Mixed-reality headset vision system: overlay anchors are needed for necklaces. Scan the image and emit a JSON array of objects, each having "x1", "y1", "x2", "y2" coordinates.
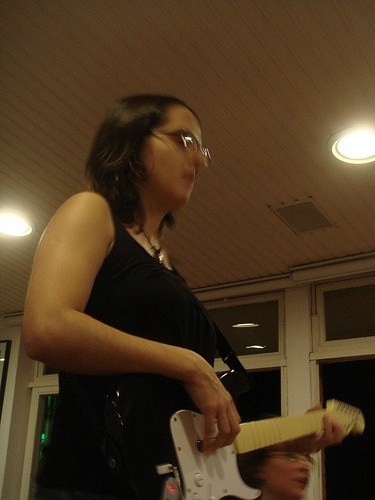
[{"x1": 143, "y1": 232, "x2": 164, "y2": 265}]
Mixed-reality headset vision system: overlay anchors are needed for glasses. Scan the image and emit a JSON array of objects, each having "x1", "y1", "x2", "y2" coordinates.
[
  {"x1": 153, "y1": 127, "x2": 211, "y2": 177},
  {"x1": 271, "y1": 451, "x2": 315, "y2": 466}
]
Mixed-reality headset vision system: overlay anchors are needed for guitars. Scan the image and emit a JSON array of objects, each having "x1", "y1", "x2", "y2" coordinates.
[{"x1": 157, "y1": 399, "x2": 366, "y2": 500}]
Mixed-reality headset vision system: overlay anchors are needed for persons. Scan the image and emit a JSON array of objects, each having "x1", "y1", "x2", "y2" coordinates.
[
  {"x1": 219, "y1": 431, "x2": 315, "y2": 500},
  {"x1": 21, "y1": 91, "x2": 343, "y2": 500}
]
